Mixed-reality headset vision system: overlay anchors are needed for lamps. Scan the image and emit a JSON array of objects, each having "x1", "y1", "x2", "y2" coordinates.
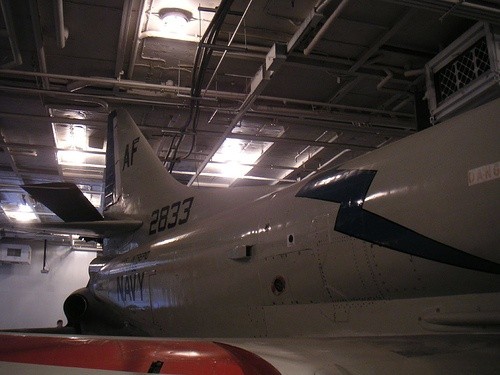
[{"x1": 157, "y1": 7, "x2": 192, "y2": 34}]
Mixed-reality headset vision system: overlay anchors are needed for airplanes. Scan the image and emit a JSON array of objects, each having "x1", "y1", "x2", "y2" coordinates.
[{"x1": 0, "y1": 96, "x2": 499, "y2": 310}]
